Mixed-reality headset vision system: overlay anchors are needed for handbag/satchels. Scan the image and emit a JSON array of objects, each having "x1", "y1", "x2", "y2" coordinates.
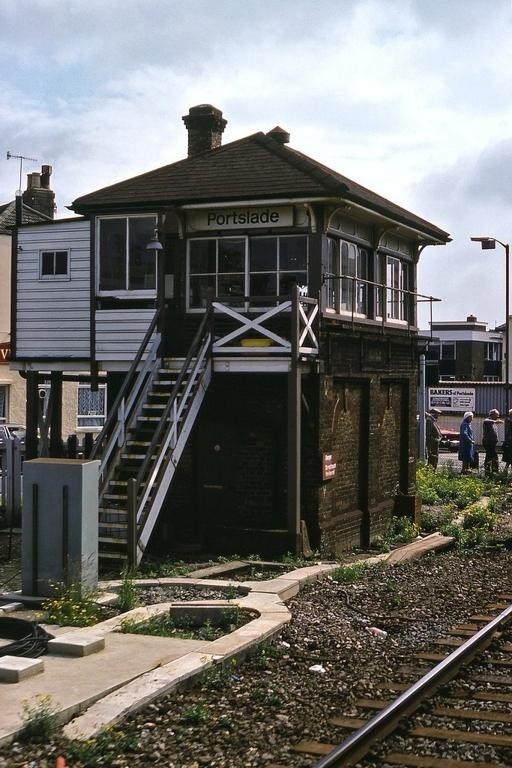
[{"x1": 470, "y1": 442, "x2": 479, "y2": 470}]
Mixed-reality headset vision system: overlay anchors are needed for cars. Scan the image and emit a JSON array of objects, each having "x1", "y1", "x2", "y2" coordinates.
[
  {"x1": 436, "y1": 424, "x2": 461, "y2": 454},
  {"x1": 0, "y1": 423, "x2": 26, "y2": 452}
]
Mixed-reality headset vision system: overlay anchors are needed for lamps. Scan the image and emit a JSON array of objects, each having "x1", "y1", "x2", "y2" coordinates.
[{"x1": 146, "y1": 227, "x2": 166, "y2": 253}]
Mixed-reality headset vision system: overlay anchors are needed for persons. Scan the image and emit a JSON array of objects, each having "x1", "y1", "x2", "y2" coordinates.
[
  {"x1": 458, "y1": 411, "x2": 477, "y2": 474},
  {"x1": 416, "y1": 412, "x2": 429, "y2": 459},
  {"x1": 482, "y1": 409, "x2": 499, "y2": 476},
  {"x1": 425, "y1": 407, "x2": 443, "y2": 473},
  {"x1": 501, "y1": 408, "x2": 512, "y2": 475}
]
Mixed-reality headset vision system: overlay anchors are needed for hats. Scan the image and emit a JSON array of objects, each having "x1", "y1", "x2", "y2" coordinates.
[
  {"x1": 430, "y1": 408, "x2": 442, "y2": 413},
  {"x1": 464, "y1": 411, "x2": 473, "y2": 419}
]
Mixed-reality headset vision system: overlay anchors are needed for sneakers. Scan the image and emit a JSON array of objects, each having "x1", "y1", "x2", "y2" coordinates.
[{"x1": 461, "y1": 470, "x2": 471, "y2": 475}]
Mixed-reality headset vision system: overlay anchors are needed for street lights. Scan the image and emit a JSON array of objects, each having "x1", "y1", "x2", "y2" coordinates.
[{"x1": 469, "y1": 236, "x2": 511, "y2": 474}]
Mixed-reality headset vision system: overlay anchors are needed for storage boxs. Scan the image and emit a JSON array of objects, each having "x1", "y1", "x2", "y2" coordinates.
[{"x1": 241, "y1": 338, "x2": 273, "y2": 356}]
